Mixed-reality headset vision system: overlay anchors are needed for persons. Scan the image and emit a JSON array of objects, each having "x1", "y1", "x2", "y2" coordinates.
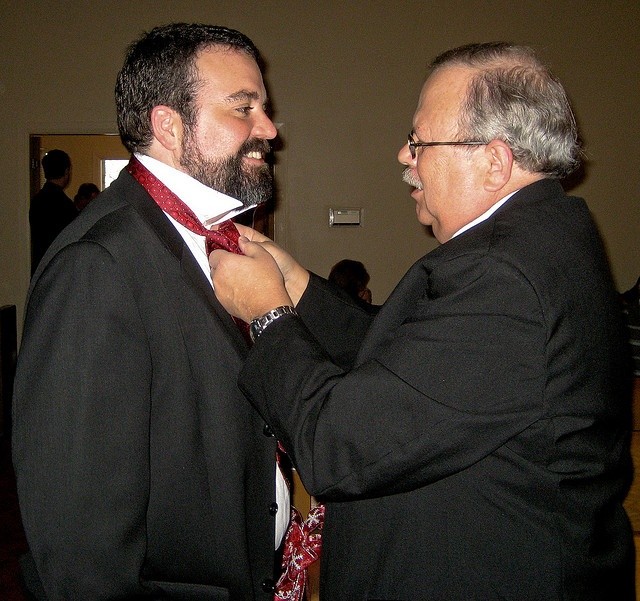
[
  {"x1": 73, "y1": 181, "x2": 101, "y2": 210},
  {"x1": 324, "y1": 257, "x2": 371, "y2": 302},
  {"x1": 29, "y1": 149, "x2": 78, "y2": 278},
  {"x1": 9, "y1": 19, "x2": 292, "y2": 600},
  {"x1": 208, "y1": 40, "x2": 634, "y2": 600}
]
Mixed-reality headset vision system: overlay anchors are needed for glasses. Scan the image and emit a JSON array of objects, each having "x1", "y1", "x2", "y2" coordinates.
[{"x1": 406, "y1": 133, "x2": 489, "y2": 160}]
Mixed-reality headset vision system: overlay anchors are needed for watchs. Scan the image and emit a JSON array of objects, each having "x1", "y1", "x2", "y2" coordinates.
[{"x1": 246, "y1": 305, "x2": 300, "y2": 340}]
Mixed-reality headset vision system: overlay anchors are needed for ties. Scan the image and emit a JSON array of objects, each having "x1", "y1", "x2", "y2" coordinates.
[
  {"x1": 273, "y1": 501, "x2": 326, "y2": 601},
  {"x1": 125, "y1": 151, "x2": 288, "y2": 463}
]
